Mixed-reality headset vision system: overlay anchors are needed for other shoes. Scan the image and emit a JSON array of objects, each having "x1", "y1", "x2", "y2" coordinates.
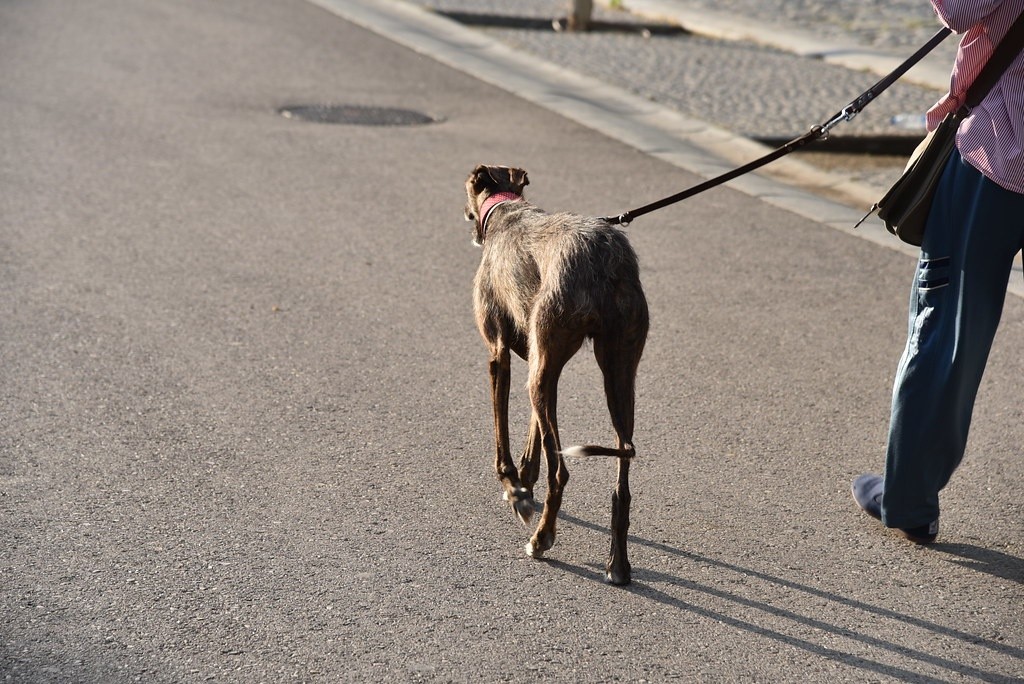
[{"x1": 852, "y1": 473, "x2": 940, "y2": 546}]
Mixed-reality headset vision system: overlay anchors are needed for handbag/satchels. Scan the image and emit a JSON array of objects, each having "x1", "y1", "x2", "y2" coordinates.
[{"x1": 855, "y1": 22, "x2": 1024, "y2": 247}]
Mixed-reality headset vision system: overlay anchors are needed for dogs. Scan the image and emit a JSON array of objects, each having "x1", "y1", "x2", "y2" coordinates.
[{"x1": 462, "y1": 163, "x2": 651, "y2": 587}]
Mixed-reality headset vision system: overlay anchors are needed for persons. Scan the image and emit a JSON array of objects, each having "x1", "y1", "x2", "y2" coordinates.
[{"x1": 848, "y1": 0, "x2": 1024, "y2": 546}]
